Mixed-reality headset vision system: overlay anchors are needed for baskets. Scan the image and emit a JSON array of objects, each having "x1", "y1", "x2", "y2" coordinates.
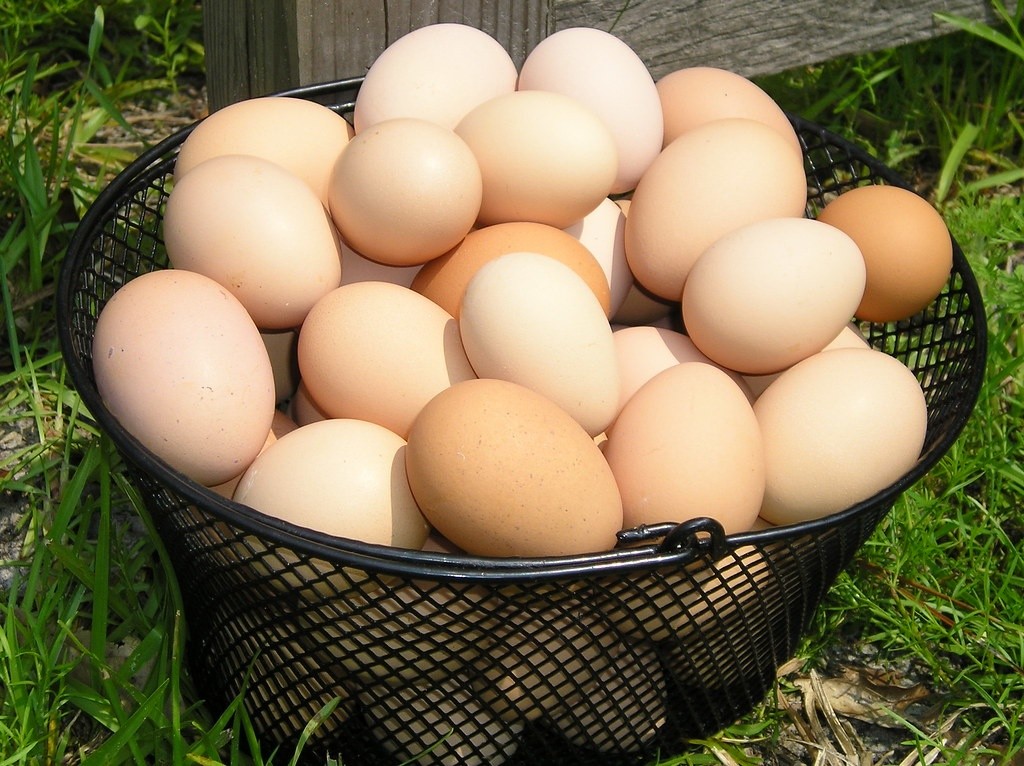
[{"x1": 56, "y1": 75, "x2": 988, "y2": 766}]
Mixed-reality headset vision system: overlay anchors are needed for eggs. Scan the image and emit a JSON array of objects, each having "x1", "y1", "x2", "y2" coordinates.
[{"x1": 89, "y1": 22, "x2": 952, "y2": 766}]
